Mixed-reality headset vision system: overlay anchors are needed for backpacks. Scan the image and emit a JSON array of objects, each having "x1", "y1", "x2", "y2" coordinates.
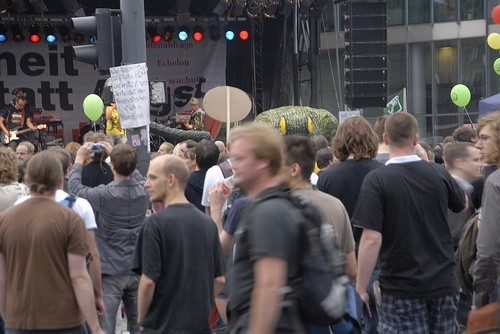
[
  {"x1": 219, "y1": 160, "x2": 241, "y2": 228},
  {"x1": 454, "y1": 206, "x2": 478, "y2": 296},
  {"x1": 241, "y1": 190, "x2": 347, "y2": 326}
]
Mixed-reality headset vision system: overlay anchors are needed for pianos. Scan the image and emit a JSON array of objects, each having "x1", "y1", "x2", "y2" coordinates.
[{"x1": 25, "y1": 107, "x2": 42, "y2": 123}]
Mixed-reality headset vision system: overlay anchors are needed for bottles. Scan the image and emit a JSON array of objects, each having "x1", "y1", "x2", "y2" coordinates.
[{"x1": 207, "y1": 174, "x2": 237, "y2": 203}]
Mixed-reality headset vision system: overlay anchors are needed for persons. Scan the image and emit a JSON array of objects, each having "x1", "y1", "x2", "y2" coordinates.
[
  {"x1": 183, "y1": 97, "x2": 205, "y2": 131},
  {"x1": 8, "y1": 90, "x2": 30, "y2": 107},
  {"x1": 0, "y1": 97, "x2": 37, "y2": 152},
  {"x1": 106, "y1": 102, "x2": 124, "y2": 146},
  {"x1": 0, "y1": 110, "x2": 500, "y2": 334}
]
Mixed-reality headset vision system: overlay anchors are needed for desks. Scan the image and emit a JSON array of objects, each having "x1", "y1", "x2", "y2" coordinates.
[{"x1": 28, "y1": 120, "x2": 63, "y2": 151}]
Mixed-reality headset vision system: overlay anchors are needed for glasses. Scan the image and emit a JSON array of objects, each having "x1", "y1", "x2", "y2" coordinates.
[{"x1": 18, "y1": 102, "x2": 25, "y2": 108}]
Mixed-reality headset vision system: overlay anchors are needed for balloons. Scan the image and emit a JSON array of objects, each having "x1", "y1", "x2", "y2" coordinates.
[
  {"x1": 492, "y1": 5, "x2": 500, "y2": 24},
  {"x1": 451, "y1": 84, "x2": 471, "y2": 110},
  {"x1": 487, "y1": 33, "x2": 500, "y2": 49},
  {"x1": 83, "y1": 93, "x2": 104, "y2": 122},
  {"x1": 493, "y1": 58, "x2": 500, "y2": 76}
]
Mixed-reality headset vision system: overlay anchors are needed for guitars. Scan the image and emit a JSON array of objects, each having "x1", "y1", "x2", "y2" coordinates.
[{"x1": 0, "y1": 123, "x2": 47, "y2": 144}]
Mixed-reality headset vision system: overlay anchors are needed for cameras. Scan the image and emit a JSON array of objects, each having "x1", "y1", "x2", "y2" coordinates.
[{"x1": 91, "y1": 143, "x2": 103, "y2": 153}]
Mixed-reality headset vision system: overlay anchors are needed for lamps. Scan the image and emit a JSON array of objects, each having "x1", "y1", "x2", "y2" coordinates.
[
  {"x1": 0, "y1": 17, "x2": 96, "y2": 44},
  {"x1": 144, "y1": 0, "x2": 312, "y2": 43}
]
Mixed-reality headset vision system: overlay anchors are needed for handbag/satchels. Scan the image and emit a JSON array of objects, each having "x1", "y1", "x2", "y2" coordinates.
[{"x1": 304, "y1": 284, "x2": 376, "y2": 334}]
